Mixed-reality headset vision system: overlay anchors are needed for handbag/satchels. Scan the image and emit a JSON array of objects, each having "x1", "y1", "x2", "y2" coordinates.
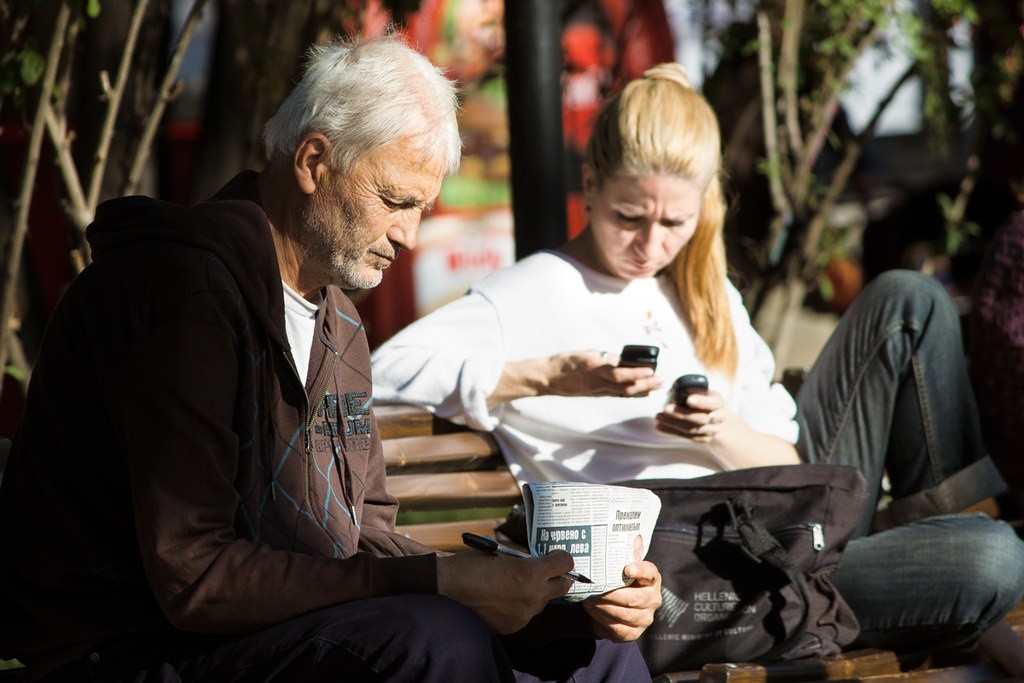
[{"x1": 494, "y1": 465, "x2": 863, "y2": 658}]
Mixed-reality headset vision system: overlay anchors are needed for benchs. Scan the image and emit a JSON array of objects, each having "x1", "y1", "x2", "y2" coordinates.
[{"x1": 1, "y1": 408, "x2": 1024, "y2": 683}]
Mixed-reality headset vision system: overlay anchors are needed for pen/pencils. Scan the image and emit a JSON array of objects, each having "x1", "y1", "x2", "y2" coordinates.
[{"x1": 461, "y1": 532, "x2": 596, "y2": 586}]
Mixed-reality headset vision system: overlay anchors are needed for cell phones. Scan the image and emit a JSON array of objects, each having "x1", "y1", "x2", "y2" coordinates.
[
  {"x1": 618, "y1": 345, "x2": 659, "y2": 371},
  {"x1": 671, "y1": 374, "x2": 709, "y2": 409}
]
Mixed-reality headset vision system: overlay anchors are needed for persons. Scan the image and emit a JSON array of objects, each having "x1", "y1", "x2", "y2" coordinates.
[
  {"x1": 633, "y1": 534, "x2": 644, "y2": 561},
  {"x1": 370, "y1": 62, "x2": 1024, "y2": 655},
  {"x1": 0, "y1": 23, "x2": 662, "y2": 683}
]
